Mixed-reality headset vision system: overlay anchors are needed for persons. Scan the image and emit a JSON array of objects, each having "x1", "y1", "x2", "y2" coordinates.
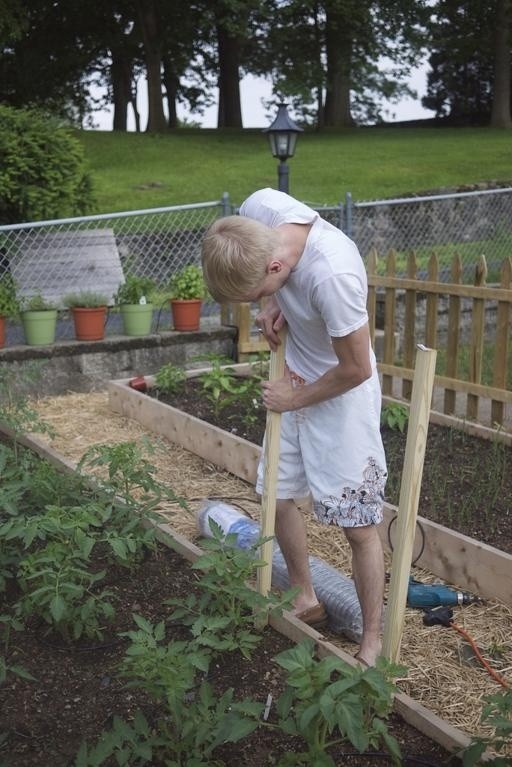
[{"x1": 201, "y1": 187, "x2": 389, "y2": 669}]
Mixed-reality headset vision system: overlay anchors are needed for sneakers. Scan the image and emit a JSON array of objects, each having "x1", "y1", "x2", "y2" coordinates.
[{"x1": 293, "y1": 600, "x2": 331, "y2": 629}]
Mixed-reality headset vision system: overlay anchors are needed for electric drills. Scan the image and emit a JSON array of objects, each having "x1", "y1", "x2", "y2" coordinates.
[{"x1": 407, "y1": 575, "x2": 488, "y2": 606}]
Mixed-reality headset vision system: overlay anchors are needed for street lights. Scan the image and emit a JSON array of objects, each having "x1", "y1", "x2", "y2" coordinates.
[{"x1": 260, "y1": 103, "x2": 305, "y2": 194}]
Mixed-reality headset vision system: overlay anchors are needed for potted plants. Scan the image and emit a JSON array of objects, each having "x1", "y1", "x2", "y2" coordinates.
[
  {"x1": 0, "y1": 271, "x2": 24, "y2": 348},
  {"x1": 167, "y1": 265, "x2": 207, "y2": 331},
  {"x1": 22, "y1": 294, "x2": 57, "y2": 345},
  {"x1": 62, "y1": 288, "x2": 108, "y2": 341},
  {"x1": 112, "y1": 273, "x2": 168, "y2": 336}
]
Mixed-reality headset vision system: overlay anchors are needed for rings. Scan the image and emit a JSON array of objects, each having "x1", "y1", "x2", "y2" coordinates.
[{"x1": 257, "y1": 328, "x2": 264, "y2": 333}]
